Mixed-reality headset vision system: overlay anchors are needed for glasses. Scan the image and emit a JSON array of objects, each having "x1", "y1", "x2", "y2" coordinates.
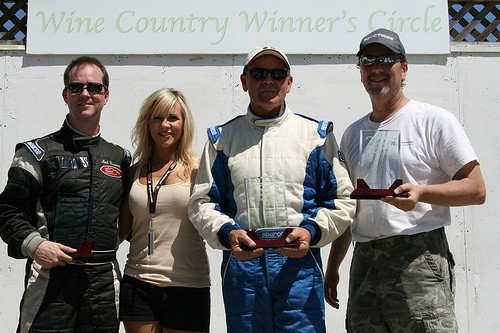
[
  {"x1": 65, "y1": 82, "x2": 108, "y2": 94},
  {"x1": 248, "y1": 68, "x2": 289, "y2": 80},
  {"x1": 360, "y1": 54, "x2": 401, "y2": 67}
]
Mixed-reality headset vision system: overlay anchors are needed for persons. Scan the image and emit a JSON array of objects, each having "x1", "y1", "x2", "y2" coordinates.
[
  {"x1": 0, "y1": 56, "x2": 135, "y2": 333},
  {"x1": 188, "y1": 45, "x2": 356, "y2": 333},
  {"x1": 120, "y1": 89, "x2": 211, "y2": 333},
  {"x1": 323, "y1": 28, "x2": 486, "y2": 333}
]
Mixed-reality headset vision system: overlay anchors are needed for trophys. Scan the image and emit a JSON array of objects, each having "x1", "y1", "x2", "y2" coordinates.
[
  {"x1": 350, "y1": 129, "x2": 402, "y2": 198},
  {"x1": 53, "y1": 191, "x2": 94, "y2": 258},
  {"x1": 243, "y1": 175, "x2": 300, "y2": 248}
]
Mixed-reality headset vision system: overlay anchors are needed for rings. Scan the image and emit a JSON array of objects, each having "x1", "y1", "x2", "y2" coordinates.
[{"x1": 231, "y1": 245, "x2": 240, "y2": 249}]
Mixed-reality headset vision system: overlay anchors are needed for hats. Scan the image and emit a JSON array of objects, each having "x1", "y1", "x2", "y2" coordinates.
[
  {"x1": 244, "y1": 45, "x2": 290, "y2": 68},
  {"x1": 356, "y1": 28, "x2": 405, "y2": 57}
]
